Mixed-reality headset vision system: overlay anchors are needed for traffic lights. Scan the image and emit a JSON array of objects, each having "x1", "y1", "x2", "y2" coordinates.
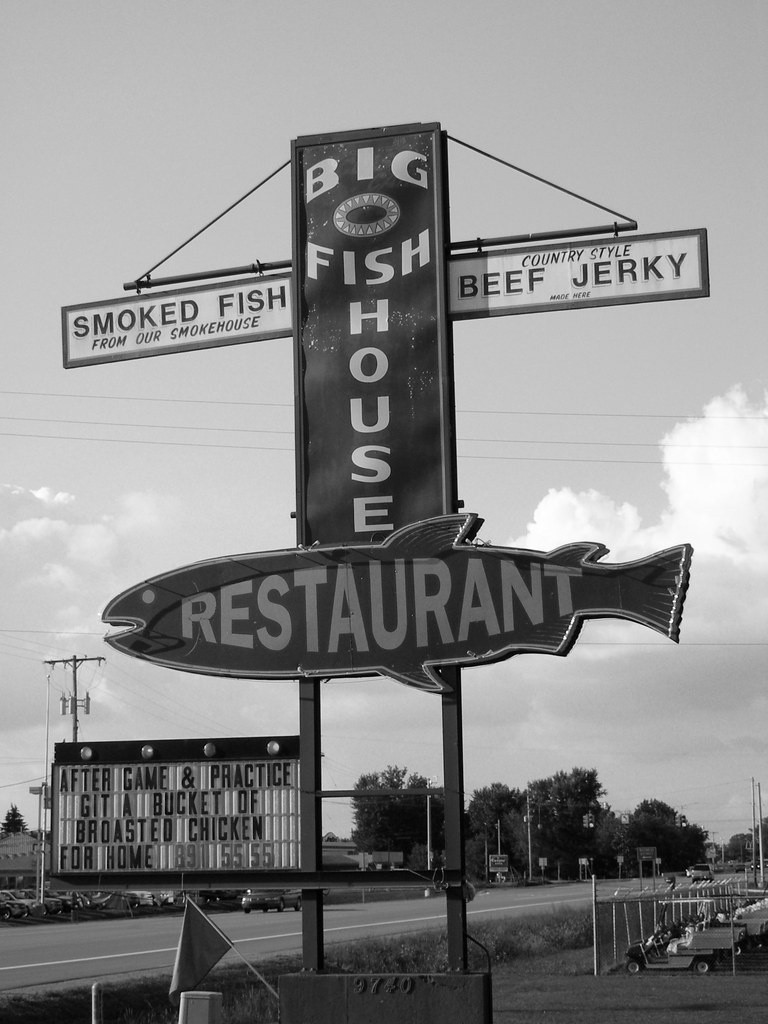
[
  {"x1": 583, "y1": 814, "x2": 595, "y2": 828},
  {"x1": 676, "y1": 814, "x2": 686, "y2": 823}
]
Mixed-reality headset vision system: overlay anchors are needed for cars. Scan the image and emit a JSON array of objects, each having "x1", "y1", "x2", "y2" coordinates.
[
  {"x1": 242, "y1": 889, "x2": 302, "y2": 913},
  {"x1": 751, "y1": 862, "x2": 760, "y2": 870},
  {"x1": 0, "y1": 889, "x2": 73, "y2": 920}
]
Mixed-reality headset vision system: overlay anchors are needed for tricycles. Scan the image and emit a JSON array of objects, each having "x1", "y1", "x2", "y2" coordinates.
[{"x1": 625, "y1": 894, "x2": 768, "y2": 974}]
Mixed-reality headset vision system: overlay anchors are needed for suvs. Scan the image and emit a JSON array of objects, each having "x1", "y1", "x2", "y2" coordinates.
[{"x1": 692, "y1": 864, "x2": 714, "y2": 883}]
